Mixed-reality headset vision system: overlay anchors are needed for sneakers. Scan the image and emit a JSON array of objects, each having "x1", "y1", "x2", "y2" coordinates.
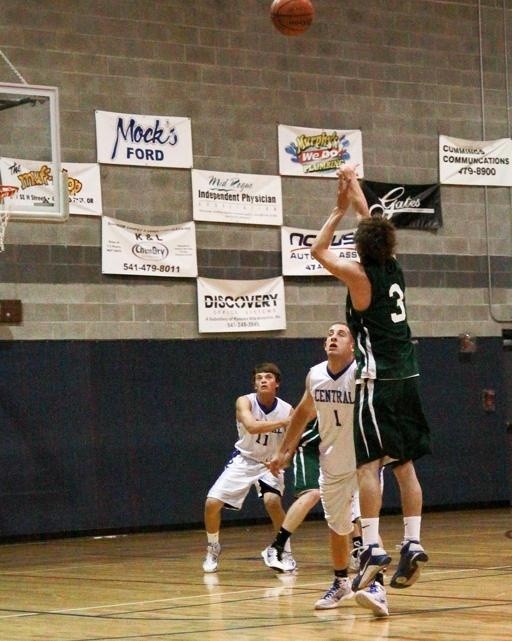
[
  {"x1": 281, "y1": 550, "x2": 297, "y2": 570},
  {"x1": 314, "y1": 577, "x2": 354, "y2": 609},
  {"x1": 352, "y1": 542, "x2": 391, "y2": 593},
  {"x1": 351, "y1": 536, "x2": 364, "y2": 570},
  {"x1": 355, "y1": 580, "x2": 388, "y2": 615},
  {"x1": 260, "y1": 544, "x2": 283, "y2": 571},
  {"x1": 390, "y1": 541, "x2": 429, "y2": 588},
  {"x1": 203, "y1": 544, "x2": 221, "y2": 572}
]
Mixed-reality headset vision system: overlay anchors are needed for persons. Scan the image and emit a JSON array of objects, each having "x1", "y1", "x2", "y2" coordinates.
[
  {"x1": 202, "y1": 363, "x2": 295, "y2": 573},
  {"x1": 310, "y1": 164, "x2": 433, "y2": 592},
  {"x1": 259, "y1": 418, "x2": 364, "y2": 573},
  {"x1": 269, "y1": 321, "x2": 389, "y2": 617}
]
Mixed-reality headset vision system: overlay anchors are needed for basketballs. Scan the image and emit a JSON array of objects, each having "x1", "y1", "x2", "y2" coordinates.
[{"x1": 270, "y1": 0, "x2": 314, "y2": 36}]
